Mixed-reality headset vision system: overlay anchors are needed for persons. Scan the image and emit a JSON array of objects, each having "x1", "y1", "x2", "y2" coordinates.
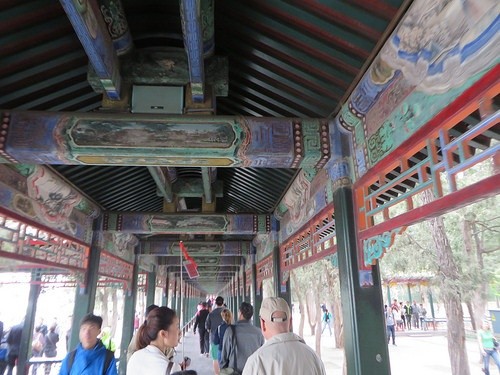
[
  {"x1": 321, "y1": 310, "x2": 332, "y2": 335},
  {"x1": 59, "y1": 314, "x2": 117, "y2": 374},
  {"x1": 8, "y1": 315, "x2": 26, "y2": 375},
  {"x1": 321, "y1": 304, "x2": 326, "y2": 312},
  {"x1": 193, "y1": 301, "x2": 212, "y2": 357},
  {"x1": 211, "y1": 308, "x2": 233, "y2": 374},
  {"x1": 32, "y1": 319, "x2": 47, "y2": 375},
  {"x1": 384, "y1": 299, "x2": 429, "y2": 331},
  {"x1": 477, "y1": 321, "x2": 500, "y2": 375},
  {"x1": 45, "y1": 322, "x2": 59, "y2": 375},
  {"x1": 197, "y1": 301, "x2": 203, "y2": 310},
  {"x1": 66, "y1": 317, "x2": 72, "y2": 351},
  {"x1": 385, "y1": 307, "x2": 396, "y2": 345},
  {"x1": 205, "y1": 296, "x2": 225, "y2": 375},
  {"x1": 219, "y1": 301, "x2": 264, "y2": 375},
  {"x1": 126, "y1": 304, "x2": 176, "y2": 363},
  {"x1": 0, "y1": 322, "x2": 11, "y2": 375},
  {"x1": 126, "y1": 306, "x2": 191, "y2": 375}
]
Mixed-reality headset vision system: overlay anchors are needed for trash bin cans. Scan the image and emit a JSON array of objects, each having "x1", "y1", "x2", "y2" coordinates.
[{"x1": 489, "y1": 310, "x2": 500, "y2": 334}]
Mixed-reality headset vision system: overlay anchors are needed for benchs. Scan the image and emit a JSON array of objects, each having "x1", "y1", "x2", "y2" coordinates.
[{"x1": 25, "y1": 357, "x2": 119, "y2": 375}]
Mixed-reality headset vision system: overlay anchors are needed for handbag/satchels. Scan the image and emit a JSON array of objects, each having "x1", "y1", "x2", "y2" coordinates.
[
  {"x1": 218, "y1": 367, "x2": 240, "y2": 375},
  {"x1": 32, "y1": 333, "x2": 42, "y2": 351}
]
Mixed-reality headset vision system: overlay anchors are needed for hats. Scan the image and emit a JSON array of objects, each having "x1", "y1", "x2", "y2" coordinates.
[{"x1": 259, "y1": 296, "x2": 291, "y2": 323}]
